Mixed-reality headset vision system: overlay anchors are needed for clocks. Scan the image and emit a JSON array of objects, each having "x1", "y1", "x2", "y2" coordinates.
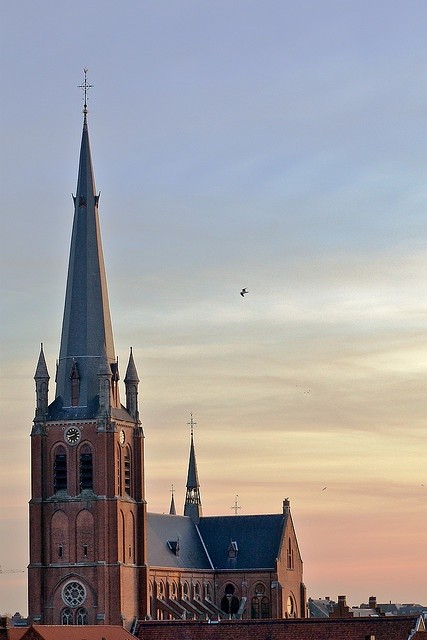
[
  {"x1": 64, "y1": 427, "x2": 82, "y2": 446},
  {"x1": 118, "y1": 429, "x2": 126, "y2": 447}
]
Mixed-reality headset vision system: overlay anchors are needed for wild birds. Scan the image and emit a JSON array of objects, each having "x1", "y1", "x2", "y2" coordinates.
[{"x1": 240, "y1": 286, "x2": 249, "y2": 297}]
